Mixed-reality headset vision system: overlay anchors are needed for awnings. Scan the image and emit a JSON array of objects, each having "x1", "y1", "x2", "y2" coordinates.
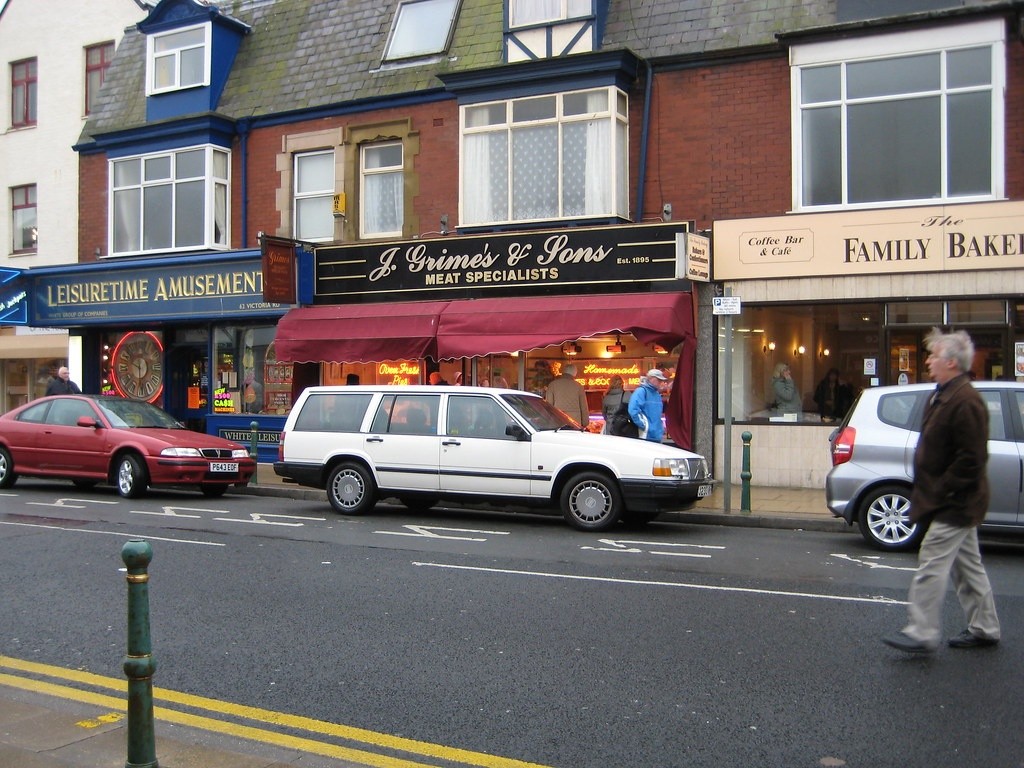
[
  {"x1": 437, "y1": 292, "x2": 696, "y2": 451},
  {"x1": 275, "y1": 292, "x2": 450, "y2": 366}
]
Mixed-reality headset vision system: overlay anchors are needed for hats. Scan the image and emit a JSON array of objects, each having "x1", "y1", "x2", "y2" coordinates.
[
  {"x1": 454, "y1": 371, "x2": 462, "y2": 383},
  {"x1": 648, "y1": 369, "x2": 667, "y2": 380}
]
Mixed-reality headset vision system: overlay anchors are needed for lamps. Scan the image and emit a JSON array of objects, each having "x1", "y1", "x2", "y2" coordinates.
[
  {"x1": 820, "y1": 348, "x2": 830, "y2": 359},
  {"x1": 794, "y1": 346, "x2": 804, "y2": 359},
  {"x1": 764, "y1": 342, "x2": 776, "y2": 358}
]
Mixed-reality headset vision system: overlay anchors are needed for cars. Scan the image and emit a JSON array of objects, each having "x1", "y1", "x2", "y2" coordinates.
[
  {"x1": 825, "y1": 381, "x2": 1024, "y2": 552},
  {"x1": 272, "y1": 385, "x2": 720, "y2": 531},
  {"x1": 0, "y1": 394, "x2": 258, "y2": 497}
]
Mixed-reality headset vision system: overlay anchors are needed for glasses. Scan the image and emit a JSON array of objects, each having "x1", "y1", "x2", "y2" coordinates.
[{"x1": 928, "y1": 354, "x2": 952, "y2": 360}]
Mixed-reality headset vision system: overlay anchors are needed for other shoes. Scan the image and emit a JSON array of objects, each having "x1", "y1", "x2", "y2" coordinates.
[
  {"x1": 947, "y1": 628, "x2": 1000, "y2": 647},
  {"x1": 880, "y1": 632, "x2": 938, "y2": 653}
]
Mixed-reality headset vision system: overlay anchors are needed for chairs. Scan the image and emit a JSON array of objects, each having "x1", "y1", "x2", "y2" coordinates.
[
  {"x1": 406, "y1": 408, "x2": 430, "y2": 434},
  {"x1": 473, "y1": 409, "x2": 494, "y2": 436}
]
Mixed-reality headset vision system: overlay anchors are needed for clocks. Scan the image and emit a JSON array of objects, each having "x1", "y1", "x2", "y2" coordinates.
[{"x1": 111, "y1": 331, "x2": 164, "y2": 404}]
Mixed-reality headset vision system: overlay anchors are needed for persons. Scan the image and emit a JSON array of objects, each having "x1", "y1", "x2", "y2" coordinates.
[
  {"x1": 771, "y1": 362, "x2": 802, "y2": 412},
  {"x1": 453, "y1": 372, "x2": 462, "y2": 386},
  {"x1": 813, "y1": 367, "x2": 841, "y2": 415},
  {"x1": 428, "y1": 372, "x2": 449, "y2": 385},
  {"x1": 601, "y1": 369, "x2": 668, "y2": 442},
  {"x1": 546, "y1": 364, "x2": 589, "y2": 427},
  {"x1": 46, "y1": 367, "x2": 84, "y2": 396},
  {"x1": 882, "y1": 330, "x2": 1001, "y2": 653}
]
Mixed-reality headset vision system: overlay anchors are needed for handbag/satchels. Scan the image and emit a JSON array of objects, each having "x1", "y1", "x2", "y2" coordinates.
[{"x1": 610, "y1": 391, "x2": 636, "y2": 436}]
